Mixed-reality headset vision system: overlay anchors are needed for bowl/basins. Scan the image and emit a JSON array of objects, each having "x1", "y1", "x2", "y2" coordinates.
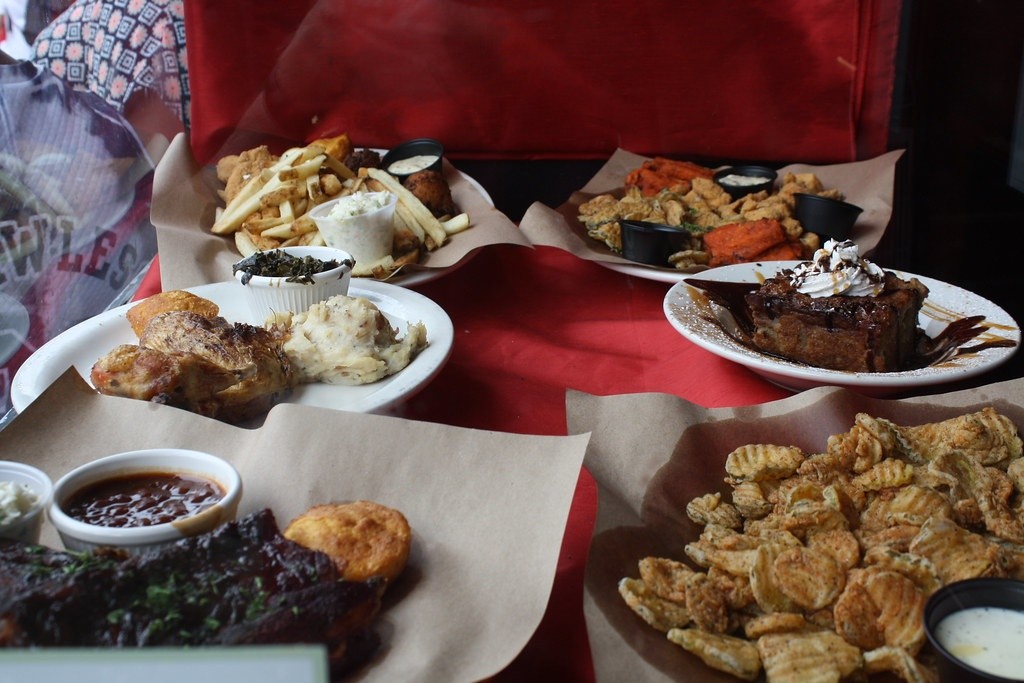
[
  {"x1": 920, "y1": 576, "x2": 1023, "y2": 683},
  {"x1": 618, "y1": 218, "x2": 690, "y2": 267},
  {"x1": 793, "y1": 192, "x2": 863, "y2": 247},
  {"x1": 309, "y1": 191, "x2": 398, "y2": 276},
  {"x1": 48, "y1": 447, "x2": 244, "y2": 558},
  {"x1": 713, "y1": 165, "x2": 777, "y2": 200},
  {"x1": 0, "y1": 460, "x2": 54, "y2": 546},
  {"x1": 381, "y1": 138, "x2": 453, "y2": 219},
  {"x1": 237, "y1": 245, "x2": 354, "y2": 321}
]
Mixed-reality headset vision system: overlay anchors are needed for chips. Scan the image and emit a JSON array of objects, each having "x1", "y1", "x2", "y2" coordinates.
[{"x1": 617, "y1": 404, "x2": 1024, "y2": 683}]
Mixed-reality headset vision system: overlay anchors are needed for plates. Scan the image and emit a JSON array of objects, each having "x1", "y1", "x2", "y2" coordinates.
[
  {"x1": 663, "y1": 262, "x2": 1021, "y2": 400},
  {"x1": 601, "y1": 263, "x2": 689, "y2": 284},
  {"x1": 10, "y1": 277, "x2": 454, "y2": 416}
]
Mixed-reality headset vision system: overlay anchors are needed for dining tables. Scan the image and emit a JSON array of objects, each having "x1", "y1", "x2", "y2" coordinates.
[{"x1": 0, "y1": 142, "x2": 1024, "y2": 683}]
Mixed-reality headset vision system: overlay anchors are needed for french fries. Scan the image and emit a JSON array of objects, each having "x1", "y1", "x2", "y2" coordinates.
[{"x1": 211, "y1": 146, "x2": 470, "y2": 273}]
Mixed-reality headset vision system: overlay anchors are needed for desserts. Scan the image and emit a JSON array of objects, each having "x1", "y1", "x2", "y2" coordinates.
[{"x1": 743, "y1": 237, "x2": 930, "y2": 374}]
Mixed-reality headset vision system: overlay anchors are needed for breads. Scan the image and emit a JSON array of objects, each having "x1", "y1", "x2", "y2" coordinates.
[
  {"x1": 128, "y1": 289, "x2": 218, "y2": 338},
  {"x1": 282, "y1": 500, "x2": 413, "y2": 586}
]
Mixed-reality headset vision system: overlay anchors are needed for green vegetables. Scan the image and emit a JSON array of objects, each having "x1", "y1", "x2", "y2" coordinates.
[{"x1": 18, "y1": 545, "x2": 304, "y2": 646}]
